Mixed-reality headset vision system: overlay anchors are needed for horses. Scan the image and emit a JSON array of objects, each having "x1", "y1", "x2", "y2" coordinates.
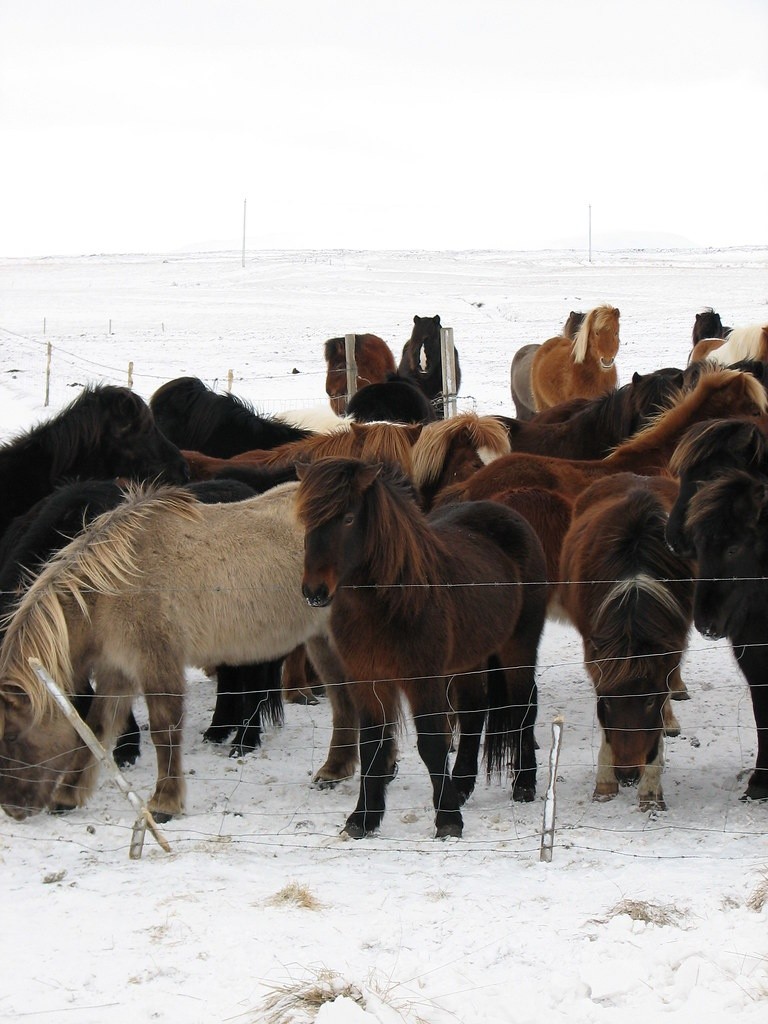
[
  {"x1": 558, "y1": 472, "x2": 690, "y2": 810},
  {"x1": 665, "y1": 418, "x2": 768, "y2": 559},
  {"x1": 688, "y1": 307, "x2": 734, "y2": 365},
  {"x1": 0, "y1": 471, "x2": 358, "y2": 825},
  {"x1": 479, "y1": 324, "x2": 768, "y2": 461},
  {"x1": 292, "y1": 454, "x2": 549, "y2": 842},
  {"x1": 688, "y1": 470, "x2": 767, "y2": 803},
  {"x1": 398, "y1": 315, "x2": 461, "y2": 420},
  {"x1": 530, "y1": 302, "x2": 619, "y2": 413},
  {"x1": 325, "y1": 334, "x2": 397, "y2": 418},
  {"x1": 511, "y1": 311, "x2": 587, "y2": 421},
  {"x1": 1, "y1": 377, "x2": 512, "y2": 772},
  {"x1": 430, "y1": 358, "x2": 768, "y2": 753}
]
